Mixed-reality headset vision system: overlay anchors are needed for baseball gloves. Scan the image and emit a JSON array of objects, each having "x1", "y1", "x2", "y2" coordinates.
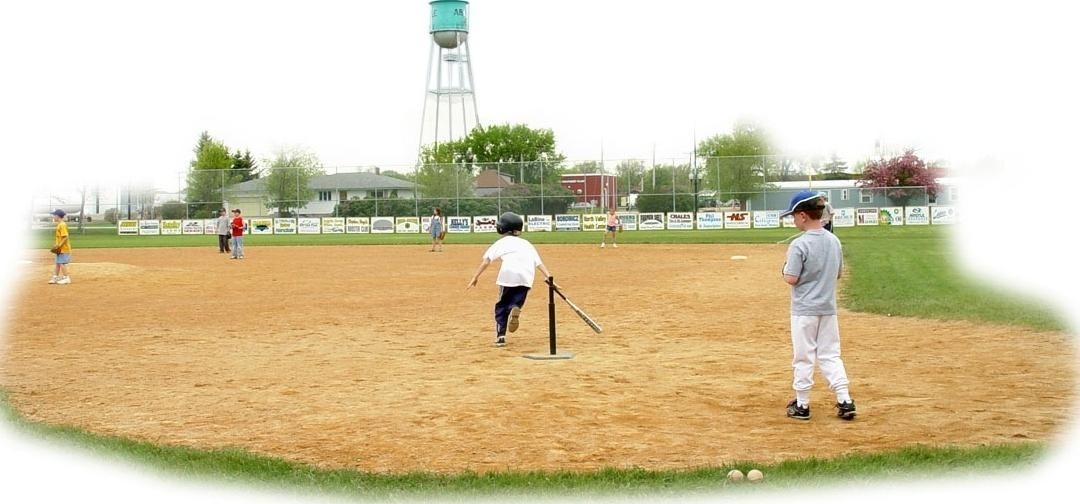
[
  {"x1": 440, "y1": 231, "x2": 445, "y2": 239},
  {"x1": 227, "y1": 231, "x2": 231, "y2": 239},
  {"x1": 50, "y1": 245, "x2": 61, "y2": 255}
]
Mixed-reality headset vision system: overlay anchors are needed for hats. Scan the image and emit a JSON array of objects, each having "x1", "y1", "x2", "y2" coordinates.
[
  {"x1": 779, "y1": 191, "x2": 821, "y2": 219},
  {"x1": 232, "y1": 209, "x2": 243, "y2": 214},
  {"x1": 50, "y1": 209, "x2": 65, "y2": 217}
]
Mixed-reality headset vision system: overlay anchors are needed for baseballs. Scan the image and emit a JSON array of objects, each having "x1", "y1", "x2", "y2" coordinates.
[
  {"x1": 747, "y1": 469, "x2": 763, "y2": 482},
  {"x1": 729, "y1": 470, "x2": 745, "y2": 483}
]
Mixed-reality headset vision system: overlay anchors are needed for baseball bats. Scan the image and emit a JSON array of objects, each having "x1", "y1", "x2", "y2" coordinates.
[{"x1": 543, "y1": 277, "x2": 602, "y2": 335}]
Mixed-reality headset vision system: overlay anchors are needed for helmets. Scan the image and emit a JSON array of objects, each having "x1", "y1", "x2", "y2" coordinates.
[{"x1": 496, "y1": 212, "x2": 523, "y2": 236}]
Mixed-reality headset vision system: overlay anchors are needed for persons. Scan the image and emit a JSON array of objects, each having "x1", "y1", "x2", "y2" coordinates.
[
  {"x1": 464, "y1": 211, "x2": 563, "y2": 346},
  {"x1": 47, "y1": 208, "x2": 73, "y2": 284},
  {"x1": 229, "y1": 208, "x2": 246, "y2": 259},
  {"x1": 600, "y1": 207, "x2": 620, "y2": 248},
  {"x1": 214, "y1": 207, "x2": 232, "y2": 254},
  {"x1": 816, "y1": 190, "x2": 835, "y2": 233},
  {"x1": 777, "y1": 191, "x2": 858, "y2": 421},
  {"x1": 425, "y1": 206, "x2": 446, "y2": 252}
]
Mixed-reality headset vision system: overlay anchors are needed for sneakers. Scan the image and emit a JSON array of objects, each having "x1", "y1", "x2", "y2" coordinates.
[
  {"x1": 494, "y1": 336, "x2": 506, "y2": 346},
  {"x1": 48, "y1": 276, "x2": 59, "y2": 284},
  {"x1": 786, "y1": 398, "x2": 812, "y2": 419},
  {"x1": 508, "y1": 306, "x2": 520, "y2": 332},
  {"x1": 836, "y1": 400, "x2": 857, "y2": 418},
  {"x1": 56, "y1": 275, "x2": 71, "y2": 285}
]
